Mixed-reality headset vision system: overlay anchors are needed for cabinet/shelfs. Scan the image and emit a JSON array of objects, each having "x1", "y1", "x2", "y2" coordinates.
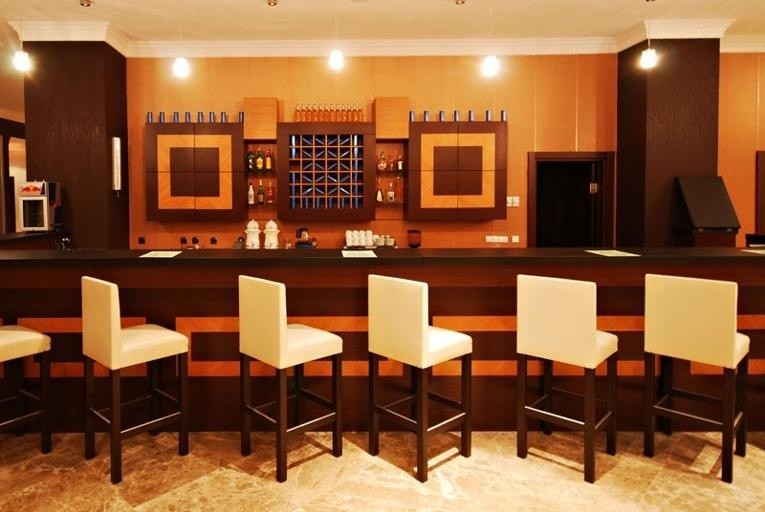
[
  {"x1": 244, "y1": 140, "x2": 278, "y2": 206},
  {"x1": 376, "y1": 138, "x2": 409, "y2": 206}
]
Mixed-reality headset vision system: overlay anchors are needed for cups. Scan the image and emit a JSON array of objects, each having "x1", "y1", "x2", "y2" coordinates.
[
  {"x1": 385, "y1": 237, "x2": 395, "y2": 247},
  {"x1": 352, "y1": 231, "x2": 359, "y2": 245},
  {"x1": 376, "y1": 237, "x2": 385, "y2": 246},
  {"x1": 407, "y1": 230, "x2": 421, "y2": 249},
  {"x1": 366, "y1": 230, "x2": 373, "y2": 245},
  {"x1": 359, "y1": 231, "x2": 366, "y2": 246},
  {"x1": 345, "y1": 231, "x2": 352, "y2": 245}
]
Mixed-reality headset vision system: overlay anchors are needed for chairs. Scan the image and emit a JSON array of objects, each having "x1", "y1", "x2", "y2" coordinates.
[
  {"x1": 642, "y1": 273, "x2": 751, "y2": 484},
  {"x1": 1, "y1": 325, "x2": 53, "y2": 454},
  {"x1": 366, "y1": 274, "x2": 474, "y2": 482},
  {"x1": 237, "y1": 275, "x2": 344, "y2": 482},
  {"x1": 81, "y1": 276, "x2": 189, "y2": 484},
  {"x1": 515, "y1": 272, "x2": 619, "y2": 483}
]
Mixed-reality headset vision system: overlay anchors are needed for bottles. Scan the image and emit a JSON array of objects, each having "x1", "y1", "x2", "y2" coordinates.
[
  {"x1": 386, "y1": 182, "x2": 395, "y2": 200},
  {"x1": 396, "y1": 176, "x2": 404, "y2": 202},
  {"x1": 247, "y1": 145, "x2": 272, "y2": 170},
  {"x1": 376, "y1": 183, "x2": 384, "y2": 202},
  {"x1": 294, "y1": 103, "x2": 363, "y2": 122},
  {"x1": 266, "y1": 180, "x2": 274, "y2": 203},
  {"x1": 248, "y1": 182, "x2": 255, "y2": 205},
  {"x1": 375, "y1": 151, "x2": 403, "y2": 172},
  {"x1": 257, "y1": 180, "x2": 264, "y2": 204}
]
